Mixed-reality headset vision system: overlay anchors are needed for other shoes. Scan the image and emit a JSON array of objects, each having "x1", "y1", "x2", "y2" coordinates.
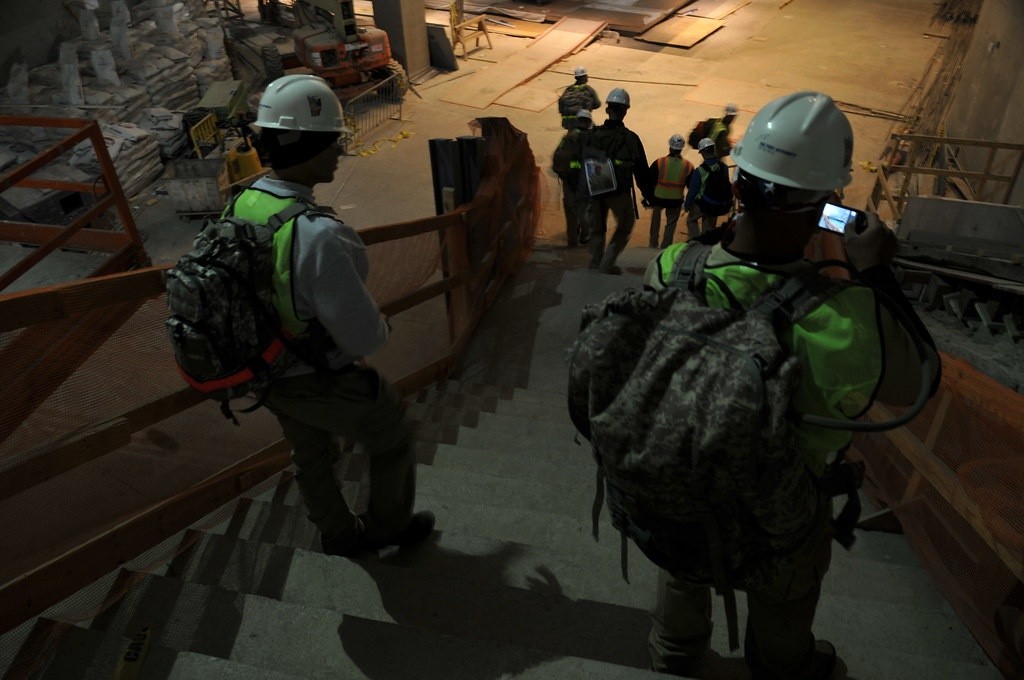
[
  {"x1": 376, "y1": 509, "x2": 436, "y2": 568},
  {"x1": 323, "y1": 510, "x2": 374, "y2": 559},
  {"x1": 801, "y1": 639, "x2": 839, "y2": 680},
  {"x1": 567, "y1": 239, "x2": 589, "y2": 248},
  {"x1": 588, "y1": 262, "x2": 599, "y2": 271},
  {"x1": 609, "y1": 265, "x2": 622, "y2": 275}
]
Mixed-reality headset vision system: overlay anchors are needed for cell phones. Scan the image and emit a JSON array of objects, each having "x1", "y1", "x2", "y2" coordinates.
[{"x1": 817, "y1": 202, "x2": 866, "y2": 236}]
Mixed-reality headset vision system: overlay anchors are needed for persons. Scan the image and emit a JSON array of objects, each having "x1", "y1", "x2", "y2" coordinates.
[
  {"x1": 642, "y1": 91, "x2": 942, "y2": 680},
  {"x1": 553, "y1": 109, "x2": 592, "y2": 247},
  {"x1": 220, "y1": 75, "x2": 436, "y2": 556},
  {"x1": 575, "y1": 88, "x2": 657, "y2": 275},
  {"x1": 648, "y1": 134, "x2": 695, "y2": 248},
  {"x1": 561, "y1": 66, "x2": 601, "y2": 132},
  {"x1": 708, "y1": 106, "x2": 738, "y2": 157},
  {"x1": 684, "y1": 138, "x2": 729, "y2": 238}
]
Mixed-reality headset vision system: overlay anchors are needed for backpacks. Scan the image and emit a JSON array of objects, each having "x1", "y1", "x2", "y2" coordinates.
[
  {"x1": 562, "y1": 240, "x2": 853, "y2": 656},
  {"x1": 692, "y1": 161, "x2": 736, "y2": 216},
  {"x1": 164, "y1": 185, "x2": 342, "y2": 427},
  {"x1": 557, "y1": 84, "x2": 593, "y2": 116},
  {"x1": 687, "y1": 118, "x2": 723, "y2": 150},
  {"x1": 576, "y1": 124, "x2": 641, "y2": 221}
]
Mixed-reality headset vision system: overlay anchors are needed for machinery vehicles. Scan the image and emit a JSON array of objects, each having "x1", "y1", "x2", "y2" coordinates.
[{"x1": 292, "y1": 0, "x2": 409, "y2": 115}]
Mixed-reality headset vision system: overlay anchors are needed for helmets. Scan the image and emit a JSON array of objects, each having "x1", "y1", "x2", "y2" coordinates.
[
  {"x1": 723, "y1": 104, "x2": 739, "y2": 116},
  {"x1": 696, "y1": 138, "x2": 715, "y2": 154},
  {"x1": 252, "y1": 74, "x2": 353, "y2": 132},
  {"x1": 728, "y1": 91, "x2": 854, "y2": 190},
  {"x1": 575, "y1": 109, "x2": 593, "y2": 123},
  {"x1": 573, "y1": 66, "x2": 588, "y2": 76},
  {"x1": 667, "y1": 133, "x2": 686, "y2": 149},
  {"x1": 605, "y1": 86, "x2": 632, "y2": 109}
]
